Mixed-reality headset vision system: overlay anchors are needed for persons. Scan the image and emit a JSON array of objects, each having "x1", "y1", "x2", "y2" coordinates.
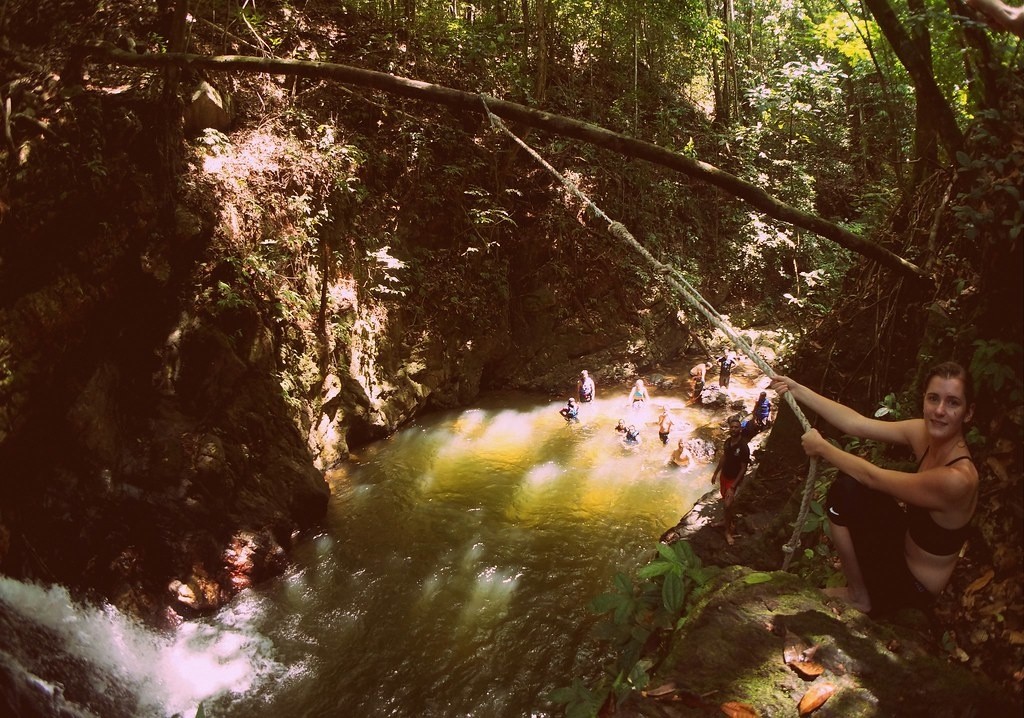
[
  {"x1": 625, "y1": 379, "x2": 652, "y2": 409},
  {"x1": 615, "y1": 418, "x2": 642, "y2": 445},
  {"x1": 752, "y1": 392, "x2": 772, "y2": 433},
  {"x1": 577, "y1": 369, "x2": 596, "y2": 403},
  {"x1": 716, "y1": 347, "x2": 737, "y2": 392},
  {"x1": 768, "y1": 360, "x2": 979, "y2": 617},
  {"x1": 670, "y1": 437, "x2": 695, "y2": 471},
  {"x1": 657, "y1": 405, "x2": 675, "y2": 445},
  {"x1": 559, "y1": 398, "x2": 581, "y2": 423},
  {"x1": 711, "y1": 420, "x2": 749, "y2": 546},
  {"x1": 689, "y1": 362, "x2": 713, "y2": 390}
]
